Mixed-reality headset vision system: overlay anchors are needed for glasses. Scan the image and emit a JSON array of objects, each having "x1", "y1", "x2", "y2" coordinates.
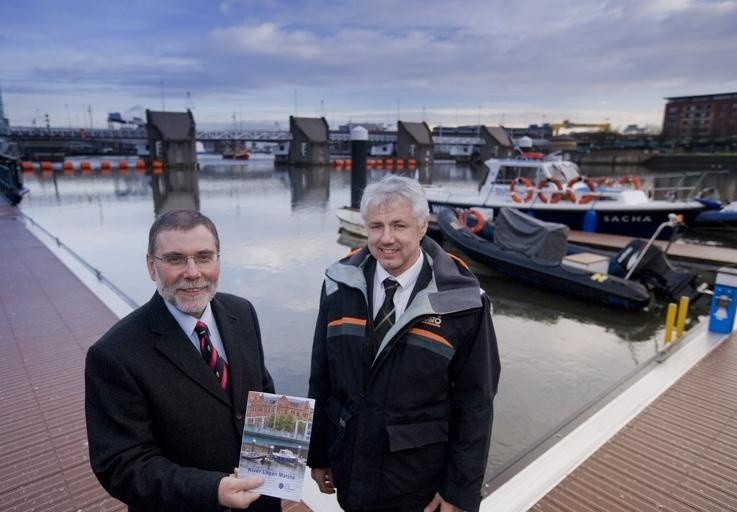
[{"x1": 150, "y1": 252, "x2": 220, "y2": 269}]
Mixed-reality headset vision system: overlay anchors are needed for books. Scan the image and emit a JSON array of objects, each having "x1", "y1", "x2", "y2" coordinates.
[{"x1": 235, "y1": 390, "x2": 315, "y2": 503}]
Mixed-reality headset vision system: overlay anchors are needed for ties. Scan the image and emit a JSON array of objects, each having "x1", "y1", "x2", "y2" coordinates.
[
  {"x1": 373, "y1": 277, "x2": 401, "y2": 350},
  {"x1": 194, "y1": 321, "x2": 229, "y2": 392}
]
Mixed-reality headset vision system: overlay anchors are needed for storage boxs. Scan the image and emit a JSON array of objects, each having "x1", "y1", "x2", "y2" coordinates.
[{"x1": 563, "y1": 252, "x2": 611, "y2": 277}]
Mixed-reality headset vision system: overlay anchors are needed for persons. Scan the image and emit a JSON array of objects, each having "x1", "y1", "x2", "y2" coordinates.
[
  {"x1": 302, "y1": 174, "x2": 501, "y2": 512},
  {"x1": 83, "y1": 207, "x2": 284, "y2": 511}
]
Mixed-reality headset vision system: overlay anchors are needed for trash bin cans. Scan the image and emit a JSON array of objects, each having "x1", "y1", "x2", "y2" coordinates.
[{"x1": 709, "y1": 266, "x2": 737, "y2": 334}]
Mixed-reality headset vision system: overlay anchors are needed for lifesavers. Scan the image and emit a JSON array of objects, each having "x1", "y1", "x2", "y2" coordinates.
[
  {"x1": 459, "y1": 209, "x2": 484, "y2": 233},
  {"x1": 537, "y1": 177, "x2": 562, "y2": 203},
  {"x1": 620, "y1": 175, "x2": 639, "y2": 190},
  {"x1": 567, "y1": 176, "x2": 594, "y2": 204},
  {"x1": 597, "y1": 178, "x2": 613, "y2": 200},
  {"x1": 510, "y1": 177, "x2": 533, "y2": 203}
]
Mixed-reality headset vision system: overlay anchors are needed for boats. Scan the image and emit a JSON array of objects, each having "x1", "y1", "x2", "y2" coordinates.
[
  {"x1": 436, "y1": 204, "x2": 688, "y2": 314},
  {"x1": 420, "y1": 134, "x2": 709, "y2": 241},
  {"x1": 269, "y1": 448, "x2": 303, "y2": 467},
  {"x1": 686, "y1": 185, "x2": 737, "y2": 231},
  {"x1": 240, "y1": 449, "x2": 270, "y2": 467},
  {"x1": 336, "y1": 205, "x2": 441, "y2": 249}
]
